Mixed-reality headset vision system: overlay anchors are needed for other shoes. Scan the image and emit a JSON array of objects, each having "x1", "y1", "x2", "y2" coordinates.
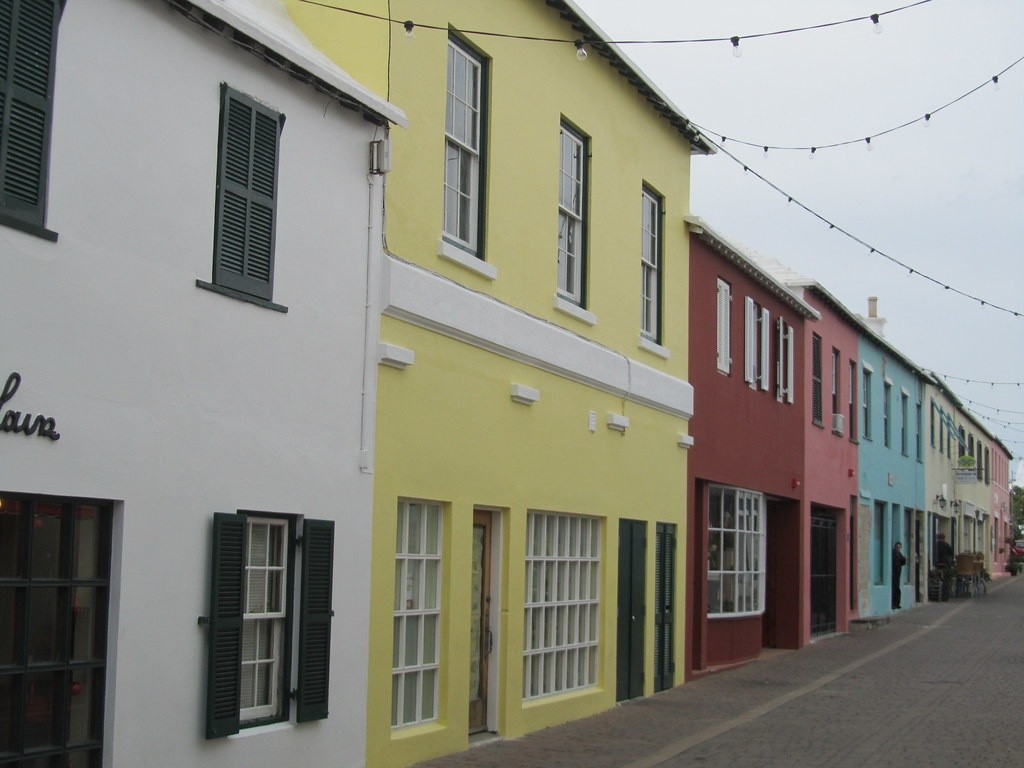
[{"x1": 892, "y1": 606, "x2": 902, "y2": 609}]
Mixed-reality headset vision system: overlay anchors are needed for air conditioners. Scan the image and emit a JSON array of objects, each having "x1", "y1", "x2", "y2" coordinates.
[{"x1": 833, "y1": 413, "x2": 844, "y2": 434}]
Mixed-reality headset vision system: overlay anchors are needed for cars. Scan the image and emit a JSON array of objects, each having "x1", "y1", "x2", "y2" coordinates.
[{"x1": 1011, "y1": 540, "x2": 1024, "y2": 561}]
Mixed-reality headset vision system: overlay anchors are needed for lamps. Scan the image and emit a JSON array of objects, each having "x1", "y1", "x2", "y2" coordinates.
[{"x1": 369, "y1": 139, "x2": 391, "y2": 176}]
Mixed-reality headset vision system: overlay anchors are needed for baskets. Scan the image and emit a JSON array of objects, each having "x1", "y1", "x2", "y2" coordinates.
[{"x1": 972, "y1": 561, "x2": 983, "y2": 571}]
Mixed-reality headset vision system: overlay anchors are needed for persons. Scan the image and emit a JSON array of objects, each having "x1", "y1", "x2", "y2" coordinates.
[
  {"x1": 938, "y1": 533, "x2": 952, "y2": 601},
  {"x1": 892, "y1": 541, "x2": 906, "y2": 609}
]
